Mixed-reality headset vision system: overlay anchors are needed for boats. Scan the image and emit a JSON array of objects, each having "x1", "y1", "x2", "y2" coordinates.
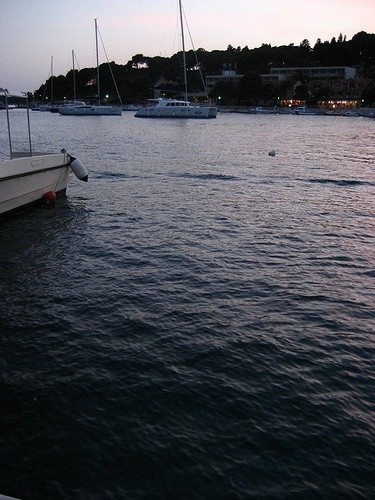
[
  {"x1": 0, "y1": 88, "x2": 89, "y2": 216},
  {"x1": 60, "y1": 19, "x2": 124, "y2": 115},
  {"x1": 47, "y1": 50, "x2": 95, "y2": 112},
  {"x1": 218, "y1": 106, "x2": 375, "y2": 117},
  {"x1": 134, "y1": 0, "x2": 218, "y2": 118},
  {"x1": 31, "y1": 81, "x2": 53, "y2": 111}
]
